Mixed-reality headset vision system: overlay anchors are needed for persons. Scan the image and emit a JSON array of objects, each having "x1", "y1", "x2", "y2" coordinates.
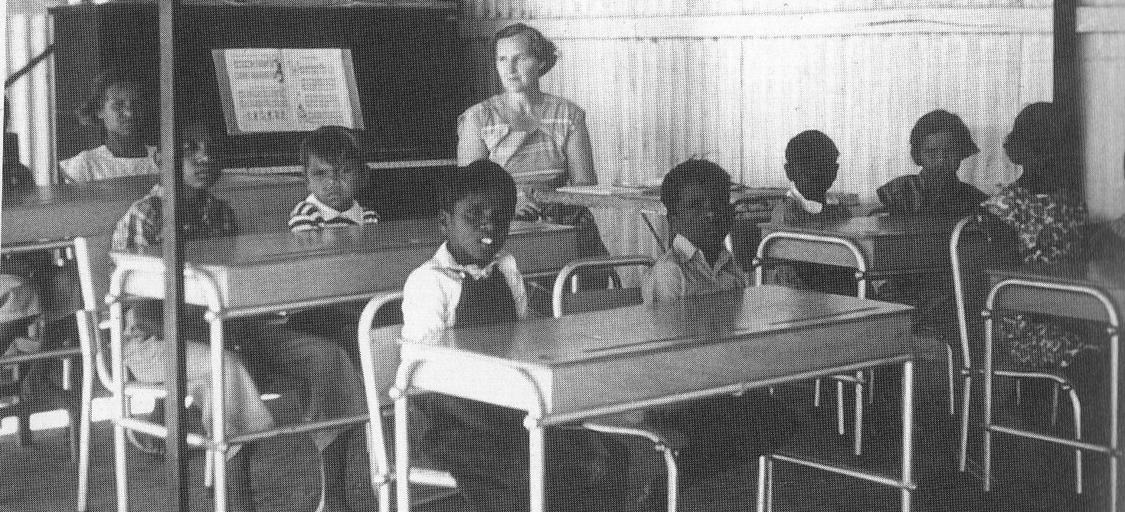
[
  {"x1": 401, "y1": 156, "x2": 632, "y2": 510},
  {"x1": 874, "y1": 103, "x2": 990, "y2": 339},
  {"x1": 51, "y1": 62, "x2": 165, "y2": 460},
  {"x1": 639, "y1": 155, "x2": 802, "y2": 511},
  {"x1": 452, "y1": 22, "x2": 624, "y2": 300},
  {"x1": 767, "y1": 125, "x2": 860, "y2": 296},
  {"x1": 3, "y1": 91, "x2": 46, "y2": 395},
  {"x1": 105, "y1": 103, "x2": 368, "y2": 512},
  {"x1": 287, "y1": 120, "x2": 383, "y2": 233},
  {"x1": 974, "y1": 103, "x2": 1119, "y2": 512}
]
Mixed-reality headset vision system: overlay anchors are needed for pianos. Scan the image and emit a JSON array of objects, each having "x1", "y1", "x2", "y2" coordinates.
[{"x1": 48, "y1": 5, "x2": 472, "y2": 221}]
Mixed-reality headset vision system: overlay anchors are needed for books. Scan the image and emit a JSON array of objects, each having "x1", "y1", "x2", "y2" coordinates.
[{"x1": 207, "y1": 45, "x2": 367, "y2": 139}]
